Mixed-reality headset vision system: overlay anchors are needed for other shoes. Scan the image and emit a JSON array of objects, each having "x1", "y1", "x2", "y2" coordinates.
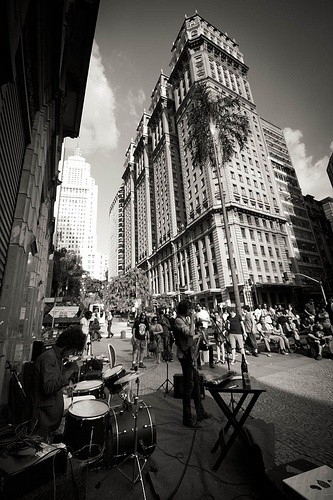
[
  {"x1": 268, "y1": 351, "x2": 271, "y2": 357},
  {"x1": 316, "y1": 353, "x2": 322, "y2": 360},
  {"x1": 281, "y1": 351, "x2": 288, "y2": 356},
  {"x1": 197, "y1": 411, "x2": 212, "y2": 420},
  {"x1": 215, "y1": 360, "x2": 220, "y2": 364},
  {"x1": 209, "y1": 365, "x2": 215, "y2": 368},
  {"x1": 252, "y1": 352, "x2": 259, "y2": 357},
  {"x1": 221, "y1": 360, "x2": 226, "y2": 364},
  {"x1": 183, "y1": 417, "x2": 202, "y2": 428},
  {"x1": 288, "y1": 348, "x2": 293, "y2": 353},
  {"x1": 197, "y1": 366, "x2": 202, "y2": 370}
]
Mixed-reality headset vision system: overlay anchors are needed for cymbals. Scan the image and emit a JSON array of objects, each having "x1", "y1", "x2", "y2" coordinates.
[{"x1": 114, "y1": 364, "x2": 158, "y2": 385}]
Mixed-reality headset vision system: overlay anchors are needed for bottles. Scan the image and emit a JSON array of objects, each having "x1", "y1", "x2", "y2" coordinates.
[{"x1": 240, "y1": 354, "x2": 250, "y2": 387}]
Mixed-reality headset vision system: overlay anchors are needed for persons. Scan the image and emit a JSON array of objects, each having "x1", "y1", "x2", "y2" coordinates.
[
  {"x1": 173, "y1": 299, "x2": 213, "y2": 428},
  {"x1": 79, "y1": 311, "x2": 92, "y2": 356},
  {"x1": 90, "y1": 318, "x2": 102, "y2": 339},
  {"x1": 26, "y1": 328, "x2": 88, "y2": 436},
  {"x1": 106, "y1": 312, "x2": 114, "y2": 338},
  {"x1": 131, "y1": 298, "x2": 333, "y2": 368}
]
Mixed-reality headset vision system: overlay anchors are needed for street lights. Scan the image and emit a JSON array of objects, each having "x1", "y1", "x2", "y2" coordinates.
[{"x1": 209, "y1": 124, "x2": 242, "y2": 315}]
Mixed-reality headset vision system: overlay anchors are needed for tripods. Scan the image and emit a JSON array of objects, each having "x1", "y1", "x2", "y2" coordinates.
[
  {"x1": 156, "y1": 359, "x2": 174, "y2": 399},
  {"x1": 95, "y1": 376, "x2": 146, "y2": 500}
]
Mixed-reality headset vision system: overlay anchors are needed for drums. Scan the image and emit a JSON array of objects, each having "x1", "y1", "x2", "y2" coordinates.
[
  {"x1": 106, "y1": 398, "x2": 157, "y2": 468},
  {"x1": 102, "y1": 364, "x2": 128, "y2": 395},
  {"x1": 68, "y1": 379, "x2": 106, "y2": 400},
  {"x1": 67, "y1": 359, "x2": 104, "y2": 384},
  {"x1": 63, "y1": 399, "x2": 111, "y2": 460}
]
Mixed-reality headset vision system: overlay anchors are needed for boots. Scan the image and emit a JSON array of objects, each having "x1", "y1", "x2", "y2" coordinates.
[
  {"x1": 130, "y1": 362, "x2": 136, "y2": 370},
  {"x1": 139, "y1": 361, "x2": 146, "y2": 368}
]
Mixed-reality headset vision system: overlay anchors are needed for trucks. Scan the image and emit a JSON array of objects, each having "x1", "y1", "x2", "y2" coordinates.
[{"x1": 44, "y1": 297, "x2": 105, "y2": 325}]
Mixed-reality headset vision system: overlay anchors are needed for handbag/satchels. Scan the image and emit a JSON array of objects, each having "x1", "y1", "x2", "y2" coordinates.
[{"x1": 148, "y1": 340, "x2": 158, "y2": 352}]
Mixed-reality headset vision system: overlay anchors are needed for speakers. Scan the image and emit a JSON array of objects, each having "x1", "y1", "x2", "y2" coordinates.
[{"x1": 173, "y1": 372, "x2": 205, "y2": 399}]
